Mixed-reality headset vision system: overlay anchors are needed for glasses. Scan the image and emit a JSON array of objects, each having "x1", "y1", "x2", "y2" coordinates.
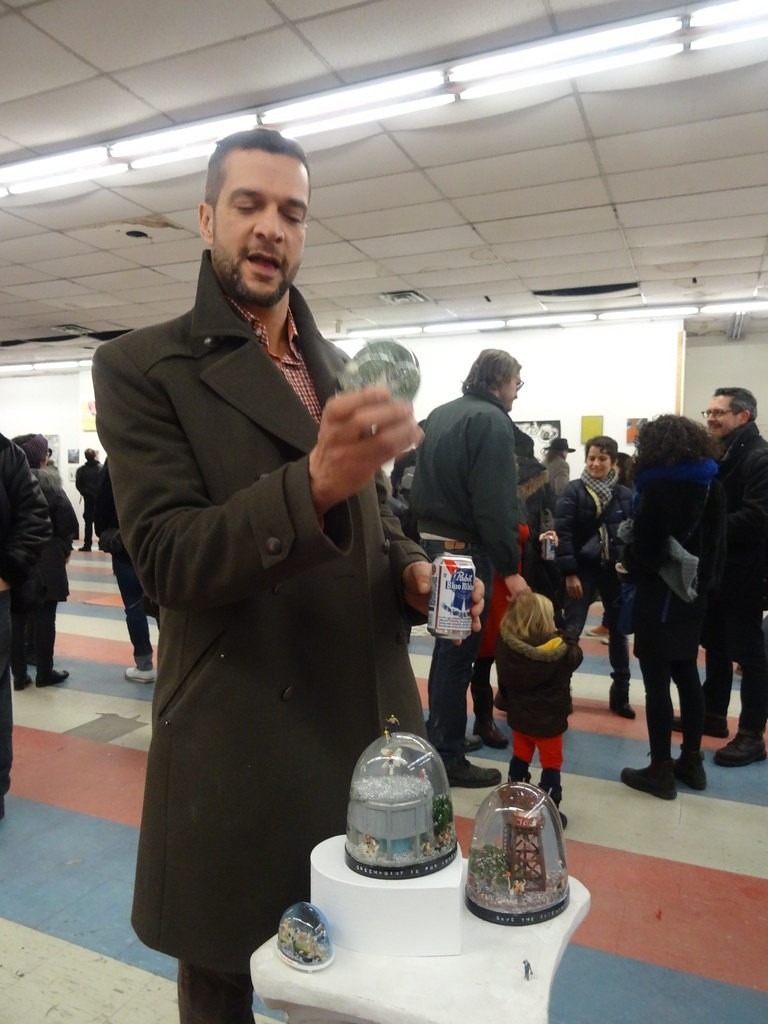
[
  {"x1": 701, "y1": 409, "x2": 739, "y2": 418},
  {"x1": 505, "y1": 379, "x2": 525, "y2": 390},
  {"x1": 43, "y1": 447, "x2": 52, "y2": 456}
]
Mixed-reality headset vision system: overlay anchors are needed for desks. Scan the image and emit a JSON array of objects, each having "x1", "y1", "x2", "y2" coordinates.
[{"x1": 251, "y1": 857, "x2": 591, "y2": 1024}]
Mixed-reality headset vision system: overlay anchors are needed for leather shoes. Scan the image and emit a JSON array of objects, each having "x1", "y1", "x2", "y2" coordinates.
[{"x1": 78, "y1": 545, "x2": 91, "y2": 552}]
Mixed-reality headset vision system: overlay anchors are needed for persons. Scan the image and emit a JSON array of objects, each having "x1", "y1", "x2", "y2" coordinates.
[
  {"x1": 0, "y1": 433, "x2": 79, "y2": 818},
  {"x1": 94, "y1": 456, "x2": 159, "y2": 682},
  {"x1": 75, "y1": 448, "x2": 102, "y2": 552},
  {"x1": 92, "y1": 132, "x2": 484, "y2": 1024},
  {"x1": 392, "y1": 351, "x2": 768, "y2": 831}
]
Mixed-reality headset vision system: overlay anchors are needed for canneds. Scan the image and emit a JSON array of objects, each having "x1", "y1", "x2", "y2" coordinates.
[
  {"x1": 427, "y1": 551, "x2": 475, "y2": 639},
  {"x1": 541, "y1": 535, "x2": 555, "y2": 560}
]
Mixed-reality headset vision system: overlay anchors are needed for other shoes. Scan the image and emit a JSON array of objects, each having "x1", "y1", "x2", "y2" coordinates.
[
  {"x1": 676, "y1": 753, "x2": 707, "y2": 789},
  {"x1": 13, "y1": 680, "x2": 32, "y2": 691},
  {"x1": 609, "y1": 702, "x2": 636, "y2": 719},
  {"x1": 674, "y1": 716, "x2": 729, "y2": 739},
  {"x1": 473, "y1": 724, "x2": 508, "y2": 749},
  {"x1": 587, "y1": 623, "x2": 609, "y2": 636},
  {"x1": 620, "y1": 767, "x2": 677, "y2": 800},
  {"x1": 444, "y1": 759, "x2": 501, "y2": 787},
  {"x1": 559, "y1": 811, "x2": 567, "y2": 829},
  {"x1": 714, "y1": 737, "x2": 766, "y2": 766},
  {"x1": 464, "y1": 735, "x2": 483, "y2": 752},
  {"x1": 36, "y1": 670, "x2": 69, "y2": 687}
]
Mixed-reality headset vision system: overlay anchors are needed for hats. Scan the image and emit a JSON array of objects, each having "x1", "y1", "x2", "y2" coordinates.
[{"x1": 542, "y1": 438, "x2": 576, "y2": 452}]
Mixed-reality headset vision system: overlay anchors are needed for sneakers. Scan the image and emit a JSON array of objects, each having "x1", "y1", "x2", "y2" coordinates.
[{"x1": 126, "y1": 668, "x2": 154, "y2": 684}]
